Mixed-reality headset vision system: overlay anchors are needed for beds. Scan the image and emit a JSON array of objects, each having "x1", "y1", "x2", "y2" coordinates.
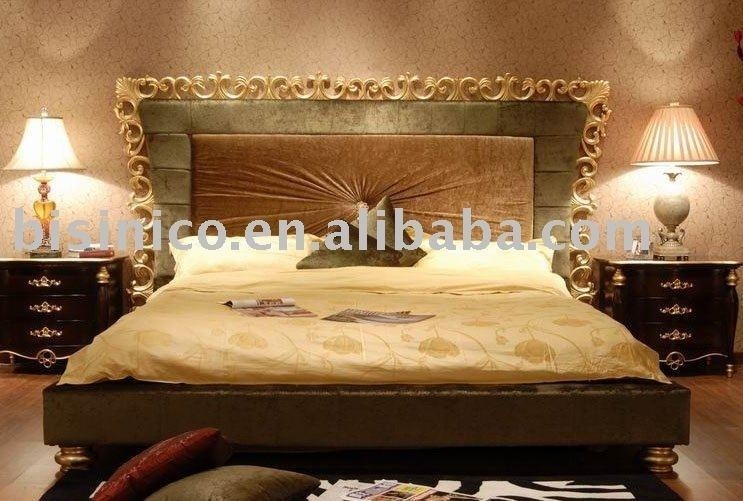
[{"x1": 42, "y1": 71, "x2": 690, "y2": 473}]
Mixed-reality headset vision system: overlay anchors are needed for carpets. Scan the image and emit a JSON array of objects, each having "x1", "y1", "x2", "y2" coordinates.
[{"x1": 36, "y1": 445, "x2": 684, "y2": 501}]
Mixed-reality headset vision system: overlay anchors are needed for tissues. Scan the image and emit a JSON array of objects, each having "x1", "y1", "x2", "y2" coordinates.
[{"x1": 79, "y1": 243, "x2": 114, "y2": 258}]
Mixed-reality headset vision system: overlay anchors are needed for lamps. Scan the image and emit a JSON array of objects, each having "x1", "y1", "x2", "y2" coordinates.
[
  {"x1": 629, "y1": 99, "x2": 720, "y2": 261},
  {"x1": 3, "y1": 105, "x2": 87, "y2": 258}
]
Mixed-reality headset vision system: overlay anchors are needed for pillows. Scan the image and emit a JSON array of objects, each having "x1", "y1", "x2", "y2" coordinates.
[
  {"x1": 143, "y1": 465, "x2": 321, "y2": 501},
  {"x1": 167, "y1": 233, "x2": 323, "y2": 277},
  {"x1": 295, "y1": 219, "x2": 402, "y2": 269},
  {"x1": 91, "y1": 427, "x2": 233, "y2": 501},
  {"x1": 415, "y1": 236, "x2": 556, "y2": 274},
  {"x1": 349, "y1": 195, "x2": 428, "y2": 266}
]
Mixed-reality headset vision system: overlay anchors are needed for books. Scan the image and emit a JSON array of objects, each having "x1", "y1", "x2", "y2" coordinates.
[
  {"x1": 321, "y1": 308, "x2": 436, "y2": 324},
  {"x1": 219, "y1": 296, "x2": 317, "y2": 320},
  {"x1": 343, "y1": 478, "x2": 514, "y2": 501}
]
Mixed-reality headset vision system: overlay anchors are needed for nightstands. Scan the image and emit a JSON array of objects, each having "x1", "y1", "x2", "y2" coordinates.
[
  {"x1": 0, "y1": 254, "x2": 131, "y2": 372},
  {"x1": 594, "y1": 257, "x2": 743, "y2": 379}
]
